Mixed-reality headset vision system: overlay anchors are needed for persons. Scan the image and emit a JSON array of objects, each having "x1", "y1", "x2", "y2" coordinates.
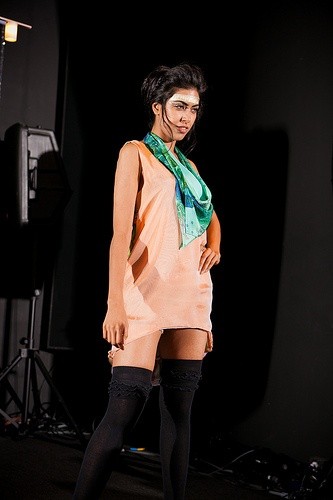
[{"x1": 72, "y1": 59, "x2": 222, "y2": 500}]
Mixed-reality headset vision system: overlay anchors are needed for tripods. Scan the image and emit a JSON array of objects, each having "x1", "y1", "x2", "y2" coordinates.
[{"x1": 0, "y1": 292, "x2": 89, "y2": 449}]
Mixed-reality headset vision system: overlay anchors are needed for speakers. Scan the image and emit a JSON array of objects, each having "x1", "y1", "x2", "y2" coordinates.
[{"x1": 0, "y1": 0, "x2": 76, "y2": 292}]
